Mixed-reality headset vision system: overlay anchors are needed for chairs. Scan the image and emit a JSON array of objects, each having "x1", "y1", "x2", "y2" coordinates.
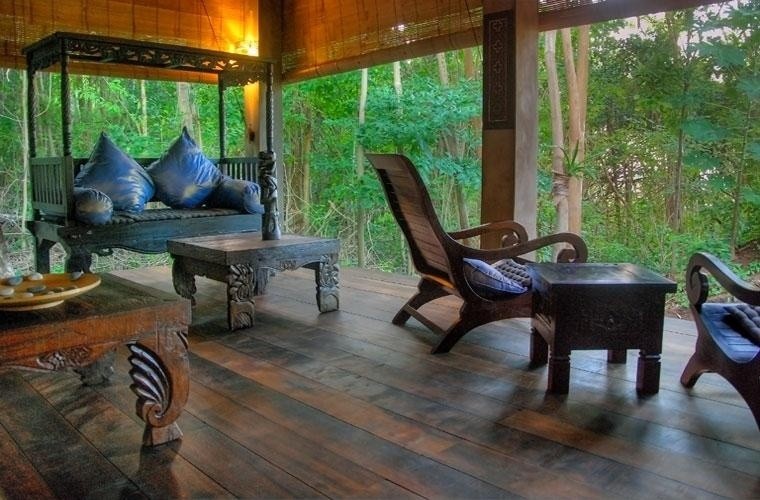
[
  {"x1": 680, "y1": 252, "x2": 759, "y2": 428},
  {"x1": 363, "y1": 151, "x2": 588, "y2": 354}
]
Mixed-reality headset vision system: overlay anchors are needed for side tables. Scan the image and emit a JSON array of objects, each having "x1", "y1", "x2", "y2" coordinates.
[{"x1": 523, "y1": 262, "x2": 677, "y2": 394}]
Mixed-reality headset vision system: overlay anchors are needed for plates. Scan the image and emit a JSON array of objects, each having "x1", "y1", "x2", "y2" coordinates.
[{"x1": 1, "y1": 272, "x2": 103, "y2": 312}]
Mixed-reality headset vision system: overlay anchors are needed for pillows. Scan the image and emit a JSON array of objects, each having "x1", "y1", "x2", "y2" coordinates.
[
  {"x1": 463, "y1": 257, "x2": 529, "y2": 295},
  {"x1": 74, "y1": 125, "x2": 263, "y2": 227}
]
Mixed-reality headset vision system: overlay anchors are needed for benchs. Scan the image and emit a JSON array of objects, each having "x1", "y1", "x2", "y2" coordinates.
[{"x1": 23, "y1": 155, "x2": 264, "y2": 274}]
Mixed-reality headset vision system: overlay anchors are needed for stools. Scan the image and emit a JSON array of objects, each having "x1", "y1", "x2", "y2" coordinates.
[{"x1": 166, "y1": 232, "x2": 341, "y2": 332}]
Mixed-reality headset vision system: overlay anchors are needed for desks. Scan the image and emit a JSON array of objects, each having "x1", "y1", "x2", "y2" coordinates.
[{"x1": 0, "y1": 271, "x2": 195, "y2": 447}]
